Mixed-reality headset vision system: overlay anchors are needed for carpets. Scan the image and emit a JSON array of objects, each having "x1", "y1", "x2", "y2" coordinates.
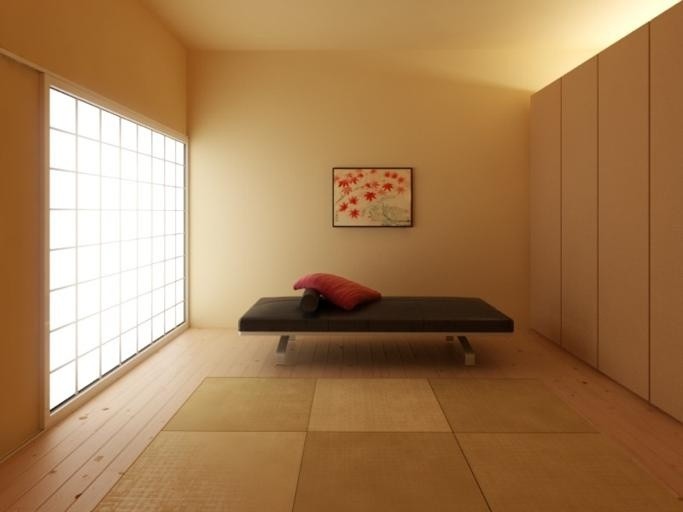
[{"x1": 88, "y1": 375, "x2": 682, "y2": 510}]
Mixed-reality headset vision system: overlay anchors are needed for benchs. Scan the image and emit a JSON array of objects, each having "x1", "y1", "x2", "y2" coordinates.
[{"x1": 237, "y1": 294, "x2": 514, "y2": 366}]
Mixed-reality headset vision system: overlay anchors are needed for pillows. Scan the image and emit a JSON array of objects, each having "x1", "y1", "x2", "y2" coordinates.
[
  {"x1": 299, "y1": 287, "x2": 319, "y2": 312},
  {"x1": 292, "y1": 272, "x2": 381, "y2": 309}
]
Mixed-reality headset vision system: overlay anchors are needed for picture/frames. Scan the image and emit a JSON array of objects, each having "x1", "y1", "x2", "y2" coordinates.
[{"x1": 331, "y1": 167, "x2": 413, "y2": 229}]
